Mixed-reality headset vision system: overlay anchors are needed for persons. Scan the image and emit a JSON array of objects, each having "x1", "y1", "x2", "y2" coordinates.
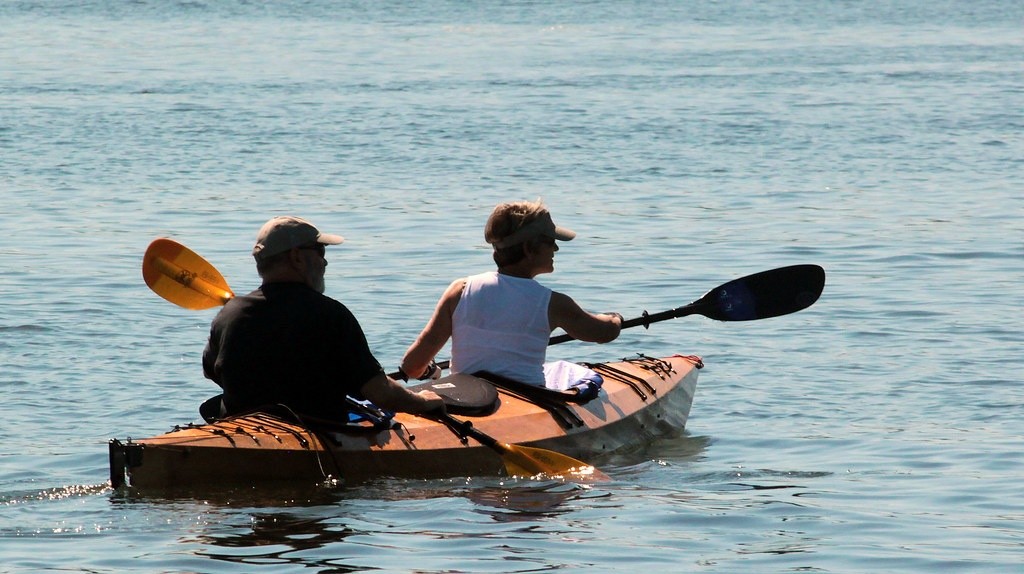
[
  {"x1": 402, "y1": 201, "x2": 623, "y2": 388},
  {"x1": 198, "y1": 216, "x2": 446, "y2": 430}
]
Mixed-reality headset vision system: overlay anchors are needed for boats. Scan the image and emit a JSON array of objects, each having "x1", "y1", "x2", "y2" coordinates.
[{"x1": 109, "y1": 355, "x2": 705, "y2": 489}]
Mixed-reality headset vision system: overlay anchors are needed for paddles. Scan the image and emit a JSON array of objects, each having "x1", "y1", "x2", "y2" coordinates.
[
  {"x1": 139, "y1": 238, "x2": 611, "y2": 488},
  {"x1": 199, "y1": 266, "x2": 827, "y2": 425}
]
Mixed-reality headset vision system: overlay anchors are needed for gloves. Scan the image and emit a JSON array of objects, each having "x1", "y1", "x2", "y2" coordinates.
[{"x1": 417, "y1": 359, "x2": 441, "y2": 380}]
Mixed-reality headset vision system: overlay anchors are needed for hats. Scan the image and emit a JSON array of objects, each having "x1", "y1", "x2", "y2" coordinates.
[
  {"x1": 492, "y1": 211, "x2": 576, "y2": 255},
  {"x1": 252, "y1": 216, "x2": 344, "y2": 262}
]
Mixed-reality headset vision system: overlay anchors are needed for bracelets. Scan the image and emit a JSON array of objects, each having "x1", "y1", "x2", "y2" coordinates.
[{"x1": 418, "y1": 360, "x2": 437, "y2": 381}]
